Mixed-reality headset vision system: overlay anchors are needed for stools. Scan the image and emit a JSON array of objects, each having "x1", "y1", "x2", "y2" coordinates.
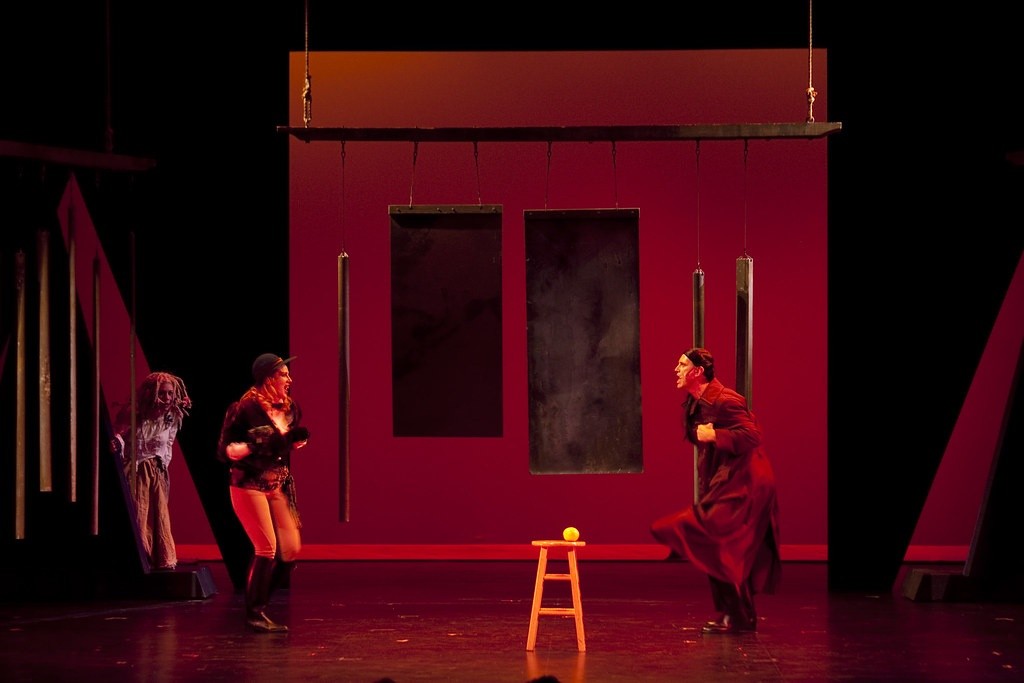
[{"x1": 526, "y1": 540, "x2": 586, "y2": 650}]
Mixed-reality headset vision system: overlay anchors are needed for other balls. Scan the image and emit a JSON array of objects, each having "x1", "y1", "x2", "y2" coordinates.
[{"x1": 563, "y1": 527, "x2": 579, "y2": 541}]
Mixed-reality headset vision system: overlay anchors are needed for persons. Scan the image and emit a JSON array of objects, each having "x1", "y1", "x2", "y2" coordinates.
[
  {"x1": 648, "y1": 348, "x2": 781, "y2": 633},
  {"x1": 111, "y1": 371, "x2": 191, "y2": 572},
  {"x1": 215, "y1": 352, "x2": 311, "y2": 632}
]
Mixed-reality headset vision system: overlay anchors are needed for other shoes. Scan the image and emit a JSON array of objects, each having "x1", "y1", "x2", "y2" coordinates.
[
  {"x1": 707, "y1": 615, "x2": 729, "y2": 627},
  {"x1": 701, "y1": 616, "x2": 736, "y2": 634},
  {"x1": 246, "y1": 612, "x2": 288, "y2": 632}
]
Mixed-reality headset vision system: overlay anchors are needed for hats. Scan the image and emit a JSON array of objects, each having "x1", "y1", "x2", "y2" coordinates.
[
  {"x1": 683, "y1": 348, "x2": 714, "y2": 382},
  {"x1": 252, "y1": 354, "x2": 298, "y2": 378}
]
